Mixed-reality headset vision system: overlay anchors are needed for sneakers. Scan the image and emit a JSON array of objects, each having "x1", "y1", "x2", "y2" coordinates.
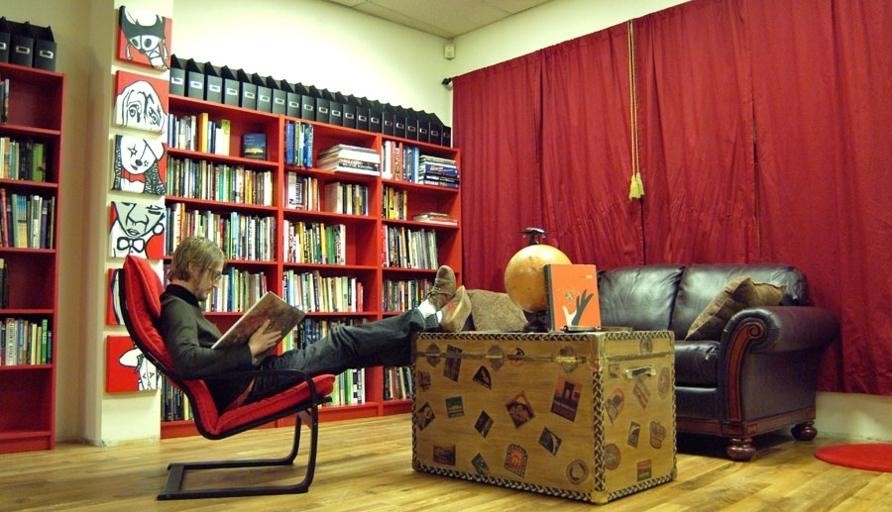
[{"x1": 428, "y1": 266, "x2": 473, "y2": 333}]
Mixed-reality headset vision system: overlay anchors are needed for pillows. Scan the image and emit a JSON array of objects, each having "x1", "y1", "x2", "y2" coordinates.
[
  {"x1": 466, "y1": 288, "x2": 528, "y2": 331},
  {"x1": 685, "y1": 276, "x2": 787, "y2": 341}
]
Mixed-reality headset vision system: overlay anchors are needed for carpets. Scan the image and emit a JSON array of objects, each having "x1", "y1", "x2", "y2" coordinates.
[{"x1": 816, "y1": 441, "x2": 892, "y2": 472}]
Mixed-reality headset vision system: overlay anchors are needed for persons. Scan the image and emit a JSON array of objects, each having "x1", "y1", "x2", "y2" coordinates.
[{"x1": 159, "y1": 234, "x2": 473, "y2": 418}]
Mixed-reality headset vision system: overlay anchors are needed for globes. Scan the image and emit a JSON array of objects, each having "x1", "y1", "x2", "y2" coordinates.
[{"x1": 504, "y1": 227, "x2": 572, "y2": 333}]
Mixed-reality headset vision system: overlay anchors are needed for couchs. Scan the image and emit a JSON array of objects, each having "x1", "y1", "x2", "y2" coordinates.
[{"x1": 464, "y1": 265, "x2": 826, "y2": 462}]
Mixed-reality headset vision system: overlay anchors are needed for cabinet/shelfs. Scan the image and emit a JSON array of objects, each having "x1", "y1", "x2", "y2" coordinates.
[
  {"x1": 0, "y1": 61, "x2": 66, "y2": 455},
  {"x1": 160, "y1": 94, "x2": 463, "y2": 437}
]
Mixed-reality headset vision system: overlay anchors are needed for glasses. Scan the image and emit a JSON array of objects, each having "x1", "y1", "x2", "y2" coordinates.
[{"x1": 198, "y1": 263, "x2": 221, "y2": 282}]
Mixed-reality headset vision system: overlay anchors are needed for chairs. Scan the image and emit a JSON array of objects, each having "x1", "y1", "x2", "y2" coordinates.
[{"x1": 118, "y1": 254, "x2": 334, "y2": 502}]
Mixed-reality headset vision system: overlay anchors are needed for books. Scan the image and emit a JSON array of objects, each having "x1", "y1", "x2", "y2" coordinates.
[
  {"x1": 282, "y1": 120, "x2": 380, "y2": 351},
  {"x1": 165, "y1": 112, "x2": 276, "y2": 313},
  {"x1": 0, "y1": 76, "x2": 56, "y2": 367},
  {"x1": 380, "y1": 140, "x2": 460, "y2": 312},
  {"x1": 383, "y1": 366, "x2": 414, "y2": 401},
  {"x1": 543, "y1": 262, "x2": 603, "y2": 333},
  {"x1": 163, "y1": 379, "x2": 193, "y2": 422},
  {"x1": 211, "y1": 290, "x2": 306, "y2": 359},
  {"x1": 316, "y1": 365, "x2": 365, "y2": 409}
]
